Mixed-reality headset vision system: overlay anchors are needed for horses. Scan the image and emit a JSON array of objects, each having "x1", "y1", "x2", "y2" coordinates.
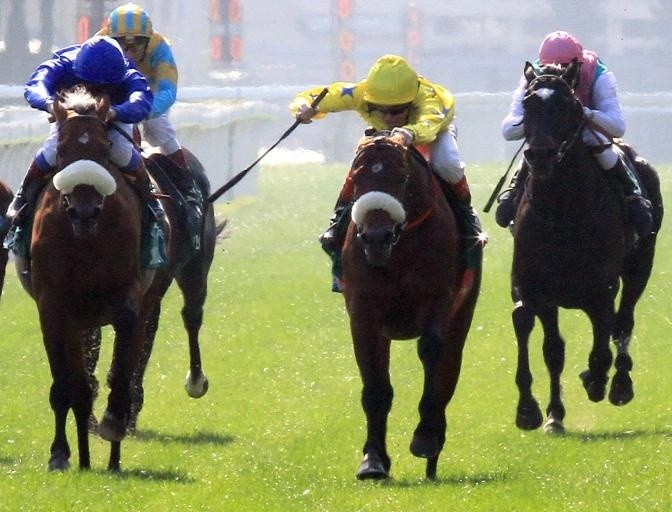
[
  {"x1": 339, "y1": 127, "x2": 483, "y2": 486},
  {"x1": 10, "y1": 82, "x2": 178, "y2": 476},
  {"x1": 80, "y1": 146, "x2": 236, "y2": 436},
  {"x1": 510, "y1": 57, "x2": 664, "y2": 438}
]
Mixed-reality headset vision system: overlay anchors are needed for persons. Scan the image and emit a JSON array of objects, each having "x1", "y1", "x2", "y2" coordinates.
[
  {"x1": 493, "y1": 30, "x2": 655, "y2": 245},
  {"x1": 92, "y1": 2, "x2": 206, "y2": 237},
  {"x1": 3, "y1": 38, "x2": 174, "y2": 270},
  {"x1": 290, "y1": 54, "x2": 490, "y2": 262}
]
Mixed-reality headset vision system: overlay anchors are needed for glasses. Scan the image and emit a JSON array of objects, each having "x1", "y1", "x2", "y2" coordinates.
[
  {"x1": 113, "y1": 36, "x2": 149, "y2": 47},
  {"x1": 366, "y1": 101, "x2": 414, "y2": 116}
]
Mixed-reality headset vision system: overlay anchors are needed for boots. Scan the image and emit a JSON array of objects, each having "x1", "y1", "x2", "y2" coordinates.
[
  {"x1": 1, "y1": 159, "x2": 51, "y2": 255},
  {"x1": 597, "y1": 146, "x2": 656, "y2": 241},
  {"x1": 318, "y1": 167, "x2": 364, "y2": 246},
  {"x1": 164, "y1": 145, "x2": 208, "y2": 223},
  {"x1": 132, "y1": 160, "x2": 172, "y2": 248},
  {"x1": 494, "y1": 156, "x2": 532, "y2": 229},
  {"x1": 445, "y1": 170, "x2": 489, "y2": 249}
]
